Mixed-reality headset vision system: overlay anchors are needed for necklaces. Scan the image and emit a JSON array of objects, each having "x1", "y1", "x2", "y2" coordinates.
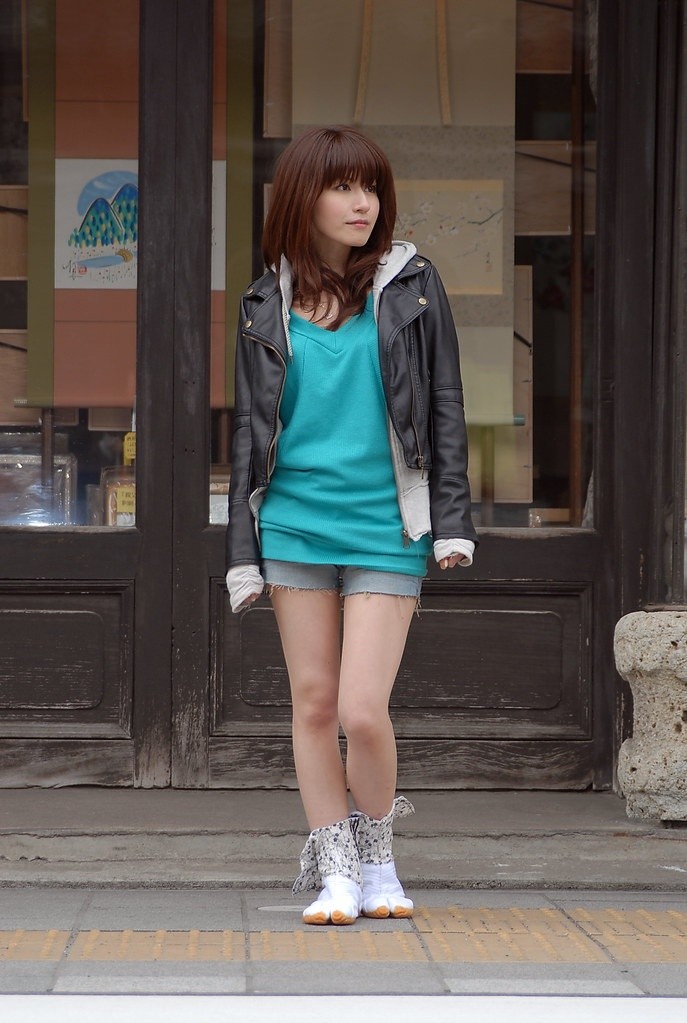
[{"x1": 319, "y1": 297, "x2": 337, "y2": 319}]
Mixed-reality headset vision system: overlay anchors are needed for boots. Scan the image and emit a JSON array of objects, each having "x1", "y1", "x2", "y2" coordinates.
[
  {"x1": 292, "y1": 818, "x2": 363, "y2": 925},
  {"x1": 348, "y1": 796, "x2": 415, "y2": 919}
]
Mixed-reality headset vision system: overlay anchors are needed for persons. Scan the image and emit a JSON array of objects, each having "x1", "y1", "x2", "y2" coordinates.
[{"x1": 223, "y1": 126, "x2": 477, "y2": 923}]
